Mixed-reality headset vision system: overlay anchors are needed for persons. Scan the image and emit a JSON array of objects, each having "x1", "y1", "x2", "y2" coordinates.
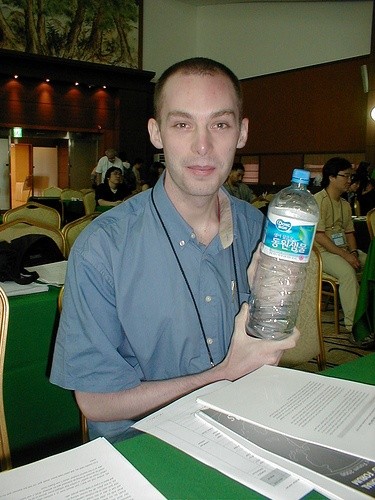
[
  {"x1": 92, "y1": 166, "x2": 132, "y2": 221},
  {"x1": 96, "y1": 148, "x2": 126, "y2": 187},
  {"x1": 221, "y1": 162, "x2": 266, "y2": 209},
  {"x1": 126, "y1": 156, "x2": 166, "y2": 196},
  {"x1": 90, "y1": 158, "x2": 101, "y2": 179},
  {"x1": 47, "y1": 57, "x2": 300, "y2": 445},
  {"x1": 313, "y1": 158, "x2": 371, "y2": 347},
  {"x1": 344, "y1": 160, "x2": 375, "y2": 216}
]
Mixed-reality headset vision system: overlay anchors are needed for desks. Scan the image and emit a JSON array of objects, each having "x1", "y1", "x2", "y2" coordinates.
[
  {"x1": 0, "y1": 261, "x2": 84, "y2": 454},
  {"x1": 0, "y1": 352, "x2": 375, "y2": 500}
]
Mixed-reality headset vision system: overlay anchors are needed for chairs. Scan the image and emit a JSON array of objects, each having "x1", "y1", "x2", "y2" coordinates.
[
  {"x1": 321, "y1": 273, "x2": 361, "y2": 334},
  {"x1": 278, "y1": 243, "x2": 324, "y2": 372},
  {"x1": 0, "y1": 219, "x2": 65, "y2": 262},
  {"x1": 3, "y1": 203, "x2": 61, "y2": 231},
  {"x1": 0, "y1": 288, "x2": 11, "y2": 472},
  {"x1": 43, "y1": 186, "x2": 96, "y2": 215},
  {"x1": 62, "y1": 214, "x2": 96, "y2": 258}
]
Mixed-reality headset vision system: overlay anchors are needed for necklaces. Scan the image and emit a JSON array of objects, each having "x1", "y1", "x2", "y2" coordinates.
[{"x1": 149, "y1": 183, "x2": 244, "y2": 367}]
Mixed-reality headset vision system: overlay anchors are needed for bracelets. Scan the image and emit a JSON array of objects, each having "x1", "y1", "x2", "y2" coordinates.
[{"x1": 350, "y1": 249, "x2": 359, "y2": 256}]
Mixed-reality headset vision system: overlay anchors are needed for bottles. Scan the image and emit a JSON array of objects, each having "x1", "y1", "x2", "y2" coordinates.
[
  {"x1": 245, "y1": 169, "x2": 319, "y2": 340},
  {"x1": 354, "y1": 193, "x2": 361, "y2": 216}
]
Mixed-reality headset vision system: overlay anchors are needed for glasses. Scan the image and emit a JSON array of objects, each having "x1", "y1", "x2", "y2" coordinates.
[{"x1": 330, "y1": 172, "x2": 353, "y2": 180}]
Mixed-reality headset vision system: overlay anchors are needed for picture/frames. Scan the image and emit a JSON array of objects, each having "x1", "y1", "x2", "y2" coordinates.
[{"x1": 0, "y1": 0, "x2": 143, "y2": 69}]
